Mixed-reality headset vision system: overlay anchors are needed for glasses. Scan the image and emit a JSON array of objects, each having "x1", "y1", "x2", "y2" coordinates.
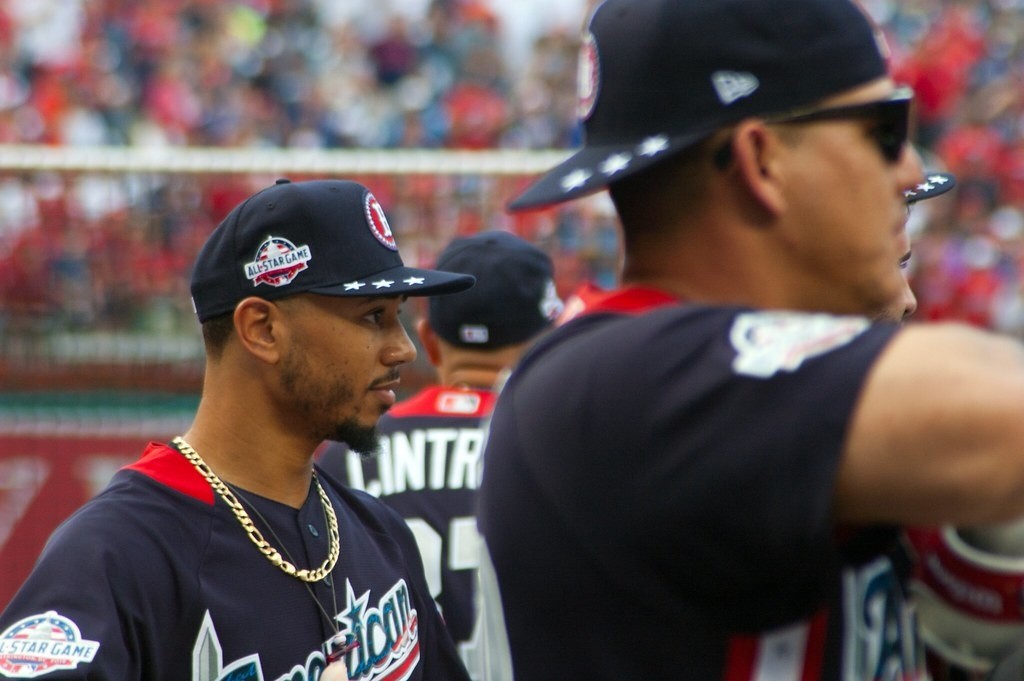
[{"x1": 711, "y1": 86, "x2": 915, "y2": 174}]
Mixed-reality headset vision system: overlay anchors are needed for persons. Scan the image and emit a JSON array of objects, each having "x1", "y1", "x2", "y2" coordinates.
[{"x1": 0, "y1": 0, "x2": 1024, "y2": 681}]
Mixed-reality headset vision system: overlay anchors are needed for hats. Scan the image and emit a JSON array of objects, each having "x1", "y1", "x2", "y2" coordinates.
[
  {"x1": 426, "y1": 226, "x2": 564, "y2": 352},
  {"x1": 505, "y1": 0, "x2": 888, "y2": 214},
  {"x1": 187, "y1": 176, "x2": 478, "y2": 321},
  {"x1": 901, "y1": 173, "x2": 955, "y2": 205}
]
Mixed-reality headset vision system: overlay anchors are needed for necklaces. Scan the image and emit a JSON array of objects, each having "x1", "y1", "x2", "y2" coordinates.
[
  {"x1": 226, "y1": 461, "x2": 360, "y2": 660},
  {"x1": 171, "y1": 436, "x2": 339, "y2": 583}
]
